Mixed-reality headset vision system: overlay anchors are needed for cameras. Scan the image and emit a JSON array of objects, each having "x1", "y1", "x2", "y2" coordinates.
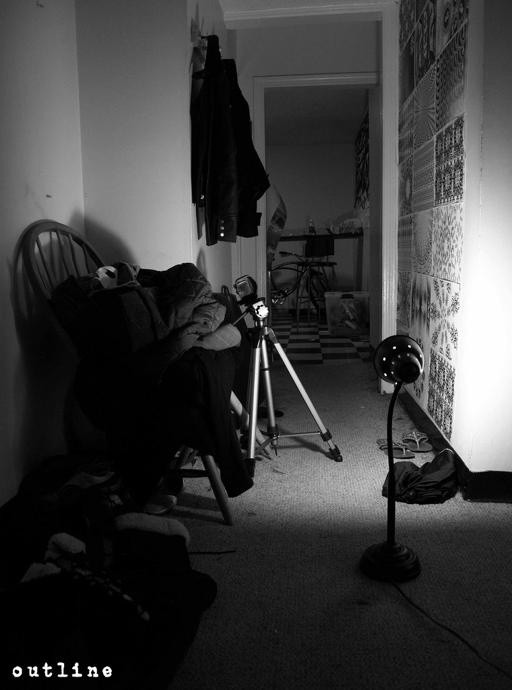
[{"x1": 231, "y1": 275, "x2": 267, "y2": 326}]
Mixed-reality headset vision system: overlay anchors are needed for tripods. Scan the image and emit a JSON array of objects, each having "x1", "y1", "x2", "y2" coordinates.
[{"x1": 244, "y1": 324, "x2": 343, "y2": 478}]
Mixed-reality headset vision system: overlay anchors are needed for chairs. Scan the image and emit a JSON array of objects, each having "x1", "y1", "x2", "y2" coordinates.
[
  {"x1": 21, "y1": 220, "x2": 273, "y2": 527},
  {"x1": 297, "y1": 237, "x2": 337, "y2": 332}
]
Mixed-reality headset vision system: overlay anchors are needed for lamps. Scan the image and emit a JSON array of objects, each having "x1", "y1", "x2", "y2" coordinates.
[{"x1": 357, "y1": 332, "x2": 425, "y2": 585}]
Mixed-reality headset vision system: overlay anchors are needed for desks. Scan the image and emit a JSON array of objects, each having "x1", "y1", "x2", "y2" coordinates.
[{"x1": 278, "y1": 227, "x2": 362, "y2": 243}]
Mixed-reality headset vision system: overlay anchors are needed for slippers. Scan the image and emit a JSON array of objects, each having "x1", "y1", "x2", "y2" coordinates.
[
  {"x1": 376, "y1": 438, "x2": 414, "y2": 459},
  {"x1": 404, "y1": 431, "x2": 434, "y2": 452}
]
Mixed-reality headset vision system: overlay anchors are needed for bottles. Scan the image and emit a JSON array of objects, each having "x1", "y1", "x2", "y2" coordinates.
[{"x1": 308, "y1": 218, "x2": 314, "y2": 234}]
